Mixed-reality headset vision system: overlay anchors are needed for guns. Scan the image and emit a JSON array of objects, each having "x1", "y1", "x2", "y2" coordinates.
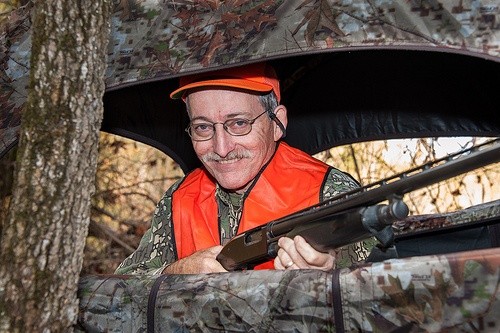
[{"x1": 214, "y1": 136, "x2": 500, "y2": 273}]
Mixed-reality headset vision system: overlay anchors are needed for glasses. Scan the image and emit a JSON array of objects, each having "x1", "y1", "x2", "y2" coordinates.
[{"x1": 183, "y1": 109, "x2": 270, "y2": 142}]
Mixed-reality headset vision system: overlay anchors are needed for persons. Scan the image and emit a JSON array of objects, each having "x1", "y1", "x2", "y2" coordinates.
[{"x1": 112, "y1": 54, "x2": 397, "y2": 294}]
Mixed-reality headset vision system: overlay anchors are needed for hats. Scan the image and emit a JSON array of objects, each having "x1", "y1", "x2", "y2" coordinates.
[{"x1": 169, "y1": 61, "x2": 281, "y2": 105}]
